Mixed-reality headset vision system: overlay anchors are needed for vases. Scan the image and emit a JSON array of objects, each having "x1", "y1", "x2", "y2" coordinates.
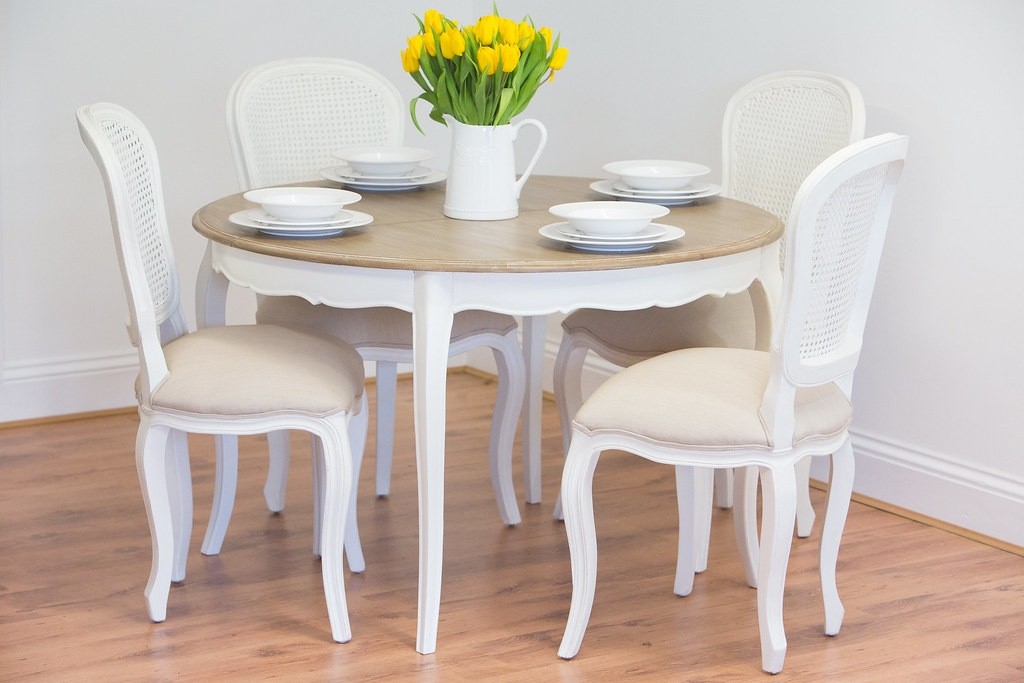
[{"x1": 445, "y1": 113, "x2": 547, "y2": 220}]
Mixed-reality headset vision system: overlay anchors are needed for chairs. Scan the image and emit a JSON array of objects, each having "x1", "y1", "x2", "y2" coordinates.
[
  {"x1": 226, "y1": 57, "x2": 527, "y2": 560},
  {"x1": 76, "y1": 101, "x2": 368, "y2": 643},
  {"x1": 550, "y1": 71, "x2": 867, "y2": 573},
  {"x1": 558, "y1": 133, "x2": 910, "y2": 673}
]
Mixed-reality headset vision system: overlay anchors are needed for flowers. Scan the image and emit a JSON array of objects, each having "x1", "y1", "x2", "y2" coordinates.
[{"x1": 399, "y1": 1, "x2": 569, "y2": 136}]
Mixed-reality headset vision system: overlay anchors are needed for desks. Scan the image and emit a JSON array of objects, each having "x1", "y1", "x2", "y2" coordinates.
[{"x1": 191, "y1": 174, "x2": 785, "y2": 656}]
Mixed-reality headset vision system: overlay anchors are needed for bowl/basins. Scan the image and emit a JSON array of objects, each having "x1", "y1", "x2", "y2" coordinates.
[
  {"x1": 243, "y1": 187, "x2": 363, "y2": 220},
  {"x1": 601, "y1": 159, "x2": 712, "y2": 189},
  {"x1": 548, "y1": 200, "x2": 671, "y2": 233},
  {"x1": 331, "y1": 147, "x2": 439, "y2": 175}
]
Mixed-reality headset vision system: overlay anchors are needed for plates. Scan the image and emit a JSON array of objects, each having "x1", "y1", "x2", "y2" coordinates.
[
  {"x1": 537, "y1": 222, "x2": 686, "y2": 253},
  {"x1": 320, "y1": 166, "x2": 448, "y2": 191},
  {"x1": 227, "y1": 209, "x2": 374, "y2": 238},
  {"x1": 611, "y1": 181, "x2": 707, "y2": 196},
  {"x1": 335, "y1": 165, "x2": 432, "y2": 183},
  {"x1": 588, "y1": 179, "x2": 720, "y2": 205},
  {"x1": 557, "y1": 223, "x2": 663, "y2": 240},
  {"x1": 246, "y1": 210, "x2": 354, "y2": 225}
]
